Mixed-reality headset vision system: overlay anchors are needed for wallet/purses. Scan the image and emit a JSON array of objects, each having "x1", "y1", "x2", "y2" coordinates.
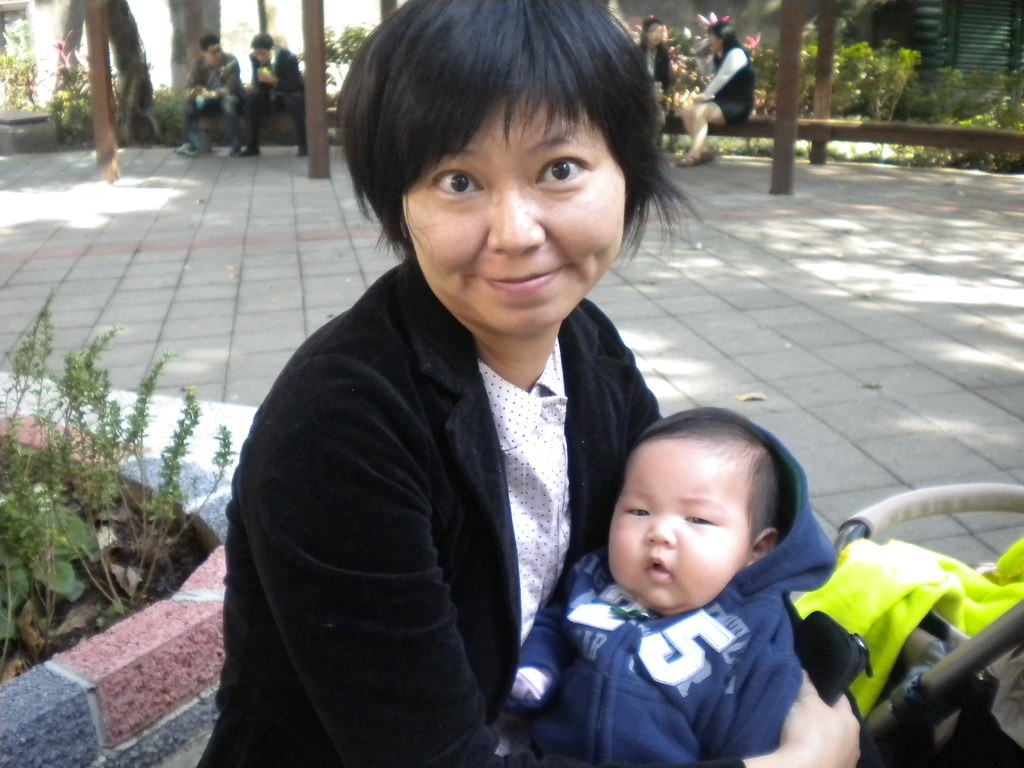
[{"x1": 792, "y1": 607, "x2": 873, "y2": 707}]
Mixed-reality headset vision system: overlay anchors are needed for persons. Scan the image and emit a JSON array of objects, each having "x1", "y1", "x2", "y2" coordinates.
[
  {"x1": 176, "y1": 34, "x2": 243, "y2": 156},
  {"x1": 238, "y1": 33, "x2": 306, "y2": 156},
  {"x1": 637, "y1": 18, "x2": 673, "y2": 145},
  {"x1": 509, "y1": 404, "x2": 837, "y2": 762},
  {"x1": 196, "y1": 0, "x2": 863, "y2": 768},
  {"x1": 679, "y1": 22, "x2": 755, "y2": 166}
]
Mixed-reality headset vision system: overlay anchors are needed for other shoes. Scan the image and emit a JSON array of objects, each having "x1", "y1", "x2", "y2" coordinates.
[
  {"x1": 297, "y1": 140, "x2": 308, "y2": 157},
  {"x1": 178, "y1": 142, "x2": 199, "y2": 156},
  {"x1": 676, "y1": 148, "x2": 715, "y2": 167},
  {"x1": 218, "y1": 145, "x2": 239, "y2": 157},
  {"x1": 241, "y1": 146, "x2": 260, "y2": 157}
]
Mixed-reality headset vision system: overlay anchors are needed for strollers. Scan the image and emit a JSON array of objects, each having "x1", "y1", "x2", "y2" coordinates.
[{"x1": 834, "y1": 483, "x2": 1024, "y2": 768}]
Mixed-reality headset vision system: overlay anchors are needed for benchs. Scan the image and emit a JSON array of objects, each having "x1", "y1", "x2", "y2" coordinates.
[{"x1": 174, "y1": 102, "x2": 1023, "y2": 150}]
